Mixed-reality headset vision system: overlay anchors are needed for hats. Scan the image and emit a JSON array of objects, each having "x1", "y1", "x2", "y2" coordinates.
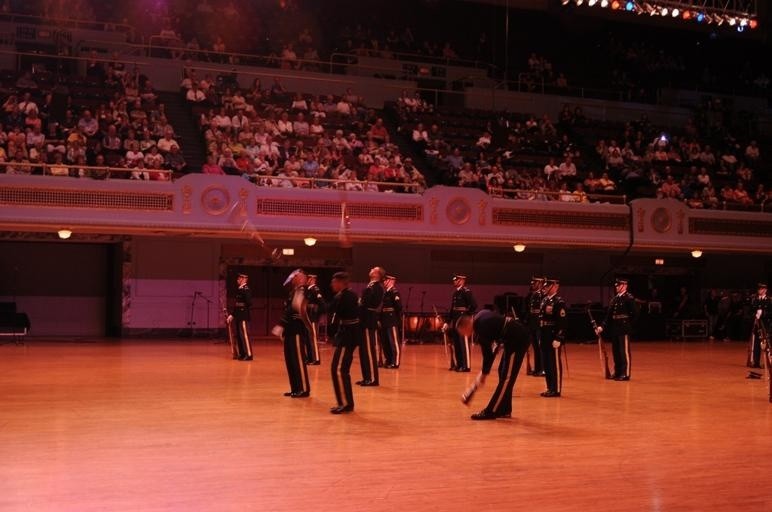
[
  {"x1": 453, "y1": 273, "x2": 467, "y2": 281},
  {"x1": 306, "y1": 272, "x2": 318, "y2": 279},
  {"x1": 530, "y1": 271, "x2": 545, "y2": 283},
  {"x1": 235, "y1": 271, "x2": 249, "y2": 277},
  {"x1": 614, "y1": 276, "x2": 628, "y2": 287},
  {"x1": 544, "y1": 273, "x2": 560, "y2": 287},
  {"x1": 757, "y1": 280, "x2": 768, "y2": 288},
  {"x1": 385, "y1": 272, "x2": 400, "y2": 280}
]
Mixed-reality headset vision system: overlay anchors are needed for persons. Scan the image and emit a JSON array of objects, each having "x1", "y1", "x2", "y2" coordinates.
[
  {"x1": 594, "y1": 277, "x2": 641, "y2": 381},
  {"x1": 0, "y1": 0, "x2": 188, "y2": 181},
  {"x1": 527, "y1": 273, "x2": 548, "y2": 377},
  {"x1": 673, "y1": 287, "x2": 689, "y2": 318},
  {"x1": 282, "y1": 269, "x2": 310, "y2": 398},
  {"x1": 356, "y1": 266, "x2": 387, "y2": 387},
  {"x1": 226, "y1": 271, "x2": 254, "y2": 362},
  {"x1": 307, "y1": 272, "x2": 363, "y2": 414},
  {"x1": 441, "y1": 272, "x2": 477, "y2": 372},
  {"x1": 302, "y1": 274, "x2": 327, "y2": 365},
  {"x1": 747, "y1": 282, "x2": 772, "y2": 368},
  {"x1": 381, "y1": 273, "x2": 403, "y2": 369},
  {"x1": 702, "y1": 284, "x2": 755, "y2": 344},
  {"x1": 455, "y1": 310, "x2": 532, "y2": 420},
  {"x1": 537, "y1": 275, "x2": 574, "y2": 398}
]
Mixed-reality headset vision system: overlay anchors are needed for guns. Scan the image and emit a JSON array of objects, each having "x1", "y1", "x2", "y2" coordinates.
[
  {"x1": 755, "y1": 316, "x2": 772, "y2": 405},
  {"x1": 220, "y1": 299, "x2": 238, "y2": 359},
  {"x1": 511, "y1": 307, "x2": 533, "y2": 375},
  {"x1": 431, "y1": 304, "x2": 457, "y2": 371},
  {"x1": 459, "y1": 341, "x2": 500, "y2": 405},
  {"x1": 587, "y1": 308, "x2": 613, "y2": 379}
]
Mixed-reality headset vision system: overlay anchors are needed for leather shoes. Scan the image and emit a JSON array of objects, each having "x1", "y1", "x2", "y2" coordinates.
[
  {"x1": 471, "y1": 410, "x2": 496, "y2": 419},
  {"x1": 356, "y1": 379, "x2": 380, "y2": 387},
  {"x1": 331, "y1": 406, "x2": 354, "y2": 414},
  {"x1": 303, "y1": 359, "x2": 320, "y2": 366},
  {"x1": 493, "y1": 411, "x2": 511, "y2": 418},
  {"x1": 284, "y1": 392, "x2": 309, "y2": 399},
  {"x1": 529, "y1": 370, "x2": 546, "y2": 376},
  {"x1": 382, "y1": 362, "x2": 399, "y2": 369},
  {"x1": 448, "y1": 366, "x2": 470, "y2": 372},
  {"x1": 238, "y1": 354, "x2": 253, "y2": 361},
  {"x1": 609, "y1": 373, "x2": 629, "y2": 381},
  {"x1": 541, "y1": 390, "x2": 561, "y2": 397}
]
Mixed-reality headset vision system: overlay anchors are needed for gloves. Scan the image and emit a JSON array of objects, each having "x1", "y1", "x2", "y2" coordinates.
[
  {"x1": 756, "y1": 309, "x2": 765, "y2": 320},
  {"x1": 225, "y1": 314, "x2": 237, "y2": 325},
  {"x1": 594, "y1": 325, "x2": 605, "y2": 335},
  {"x1": 442, "y1": 321, "x2": 451, "y2": 333},
  {"x1": 553, "y1": 339, "x2": 564, "y2": 351}
]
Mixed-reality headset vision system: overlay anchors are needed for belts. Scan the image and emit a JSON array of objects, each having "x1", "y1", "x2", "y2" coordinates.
[
  {"x1": 539, "y1": 319, "x2": 556, "y2": 327},
  {"x1": 530, "y1": 309, "x2": 542, "y2": 313},
  {"x1": 383, "y1": 307, "x2": 395, "y2": 312},
  {"x1": 339, "y1": 319, "x2": 360, "y2": 325},
  {"x1": 453, "y1": 306, "x2": 468, "y2": 311},
  {"x1": 502, "y1": 316, "x2": 514, "y2": 335},
  {"x1": 612, "y1": 314, "x2": 631, "y2": 319}
]
